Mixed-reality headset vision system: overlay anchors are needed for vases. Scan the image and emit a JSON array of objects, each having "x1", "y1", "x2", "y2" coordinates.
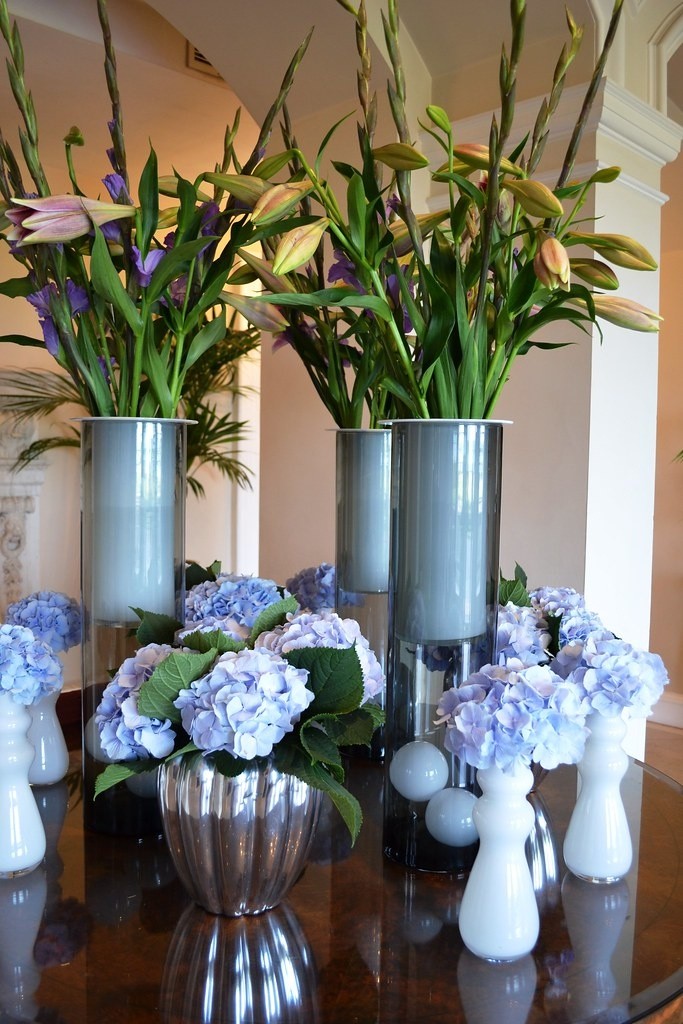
[
  {"x1": 157, "y1": 753, "x2": 326, "y2": 919},
  {"x1": 562, "y1": 712, "x2": 633, "y2": 884},
  {"x1": 27, "y1": 689, "x2": 69, "y2": 786},
  {"x1": 70, "y1": 416, "x2": 198, "y2": 837},
  {"x1": 0, "y1": 696, "x2": 47, "y2": 879},
  {"x1": 161, "y1": 902, "x2": 320, "y2": 1024},
  {"x1": 458, "y1": 761, "x2": 539, "y2": 963},
  {"x1": 324, "y1": 427, "x2": 391, "y2": 669},
  {"x1": 0, "y1": 866, "x2": 46, "y2": 1024},
  {"x1": 563, "y1": 876, "x2": 630, "y2": 1016},
  {"x1": 28, "y1": 785, "x2": 67, "y2": 909},
  {"x1": 376, "y1": 418, "x2": 513, "y2": 879},
  {"x1": 458, "y1": 950, "x2": 539, "y2": 1024},
  {"x1": 530, "y1": 759, "x2": 549, "y2": 792}
]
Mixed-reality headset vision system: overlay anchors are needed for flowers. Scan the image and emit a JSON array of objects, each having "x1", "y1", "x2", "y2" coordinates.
[
  {"x1": 93, "y1": 561, "x2": 385, "y2": 849},
  {"x1": 544, "y1": 950, "x2": 630, "y2": 1024},
  {"x1": 33, "y1": 896, "x2": 92, "y2": 968},
  {"x1": 432, "y1": 561, "x2": 670, "y2": 771},
  {"x1": 1, "y1": 1, "x2": 664, "y2": 496},
  {"x1": 0, "y1": 590, "x2": 87, "y2": 704}
]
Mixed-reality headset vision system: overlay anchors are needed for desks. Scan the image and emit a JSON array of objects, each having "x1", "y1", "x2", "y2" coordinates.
[{"x1": 0, "y1": 704, "x2": 683, "y2": 1024}]
